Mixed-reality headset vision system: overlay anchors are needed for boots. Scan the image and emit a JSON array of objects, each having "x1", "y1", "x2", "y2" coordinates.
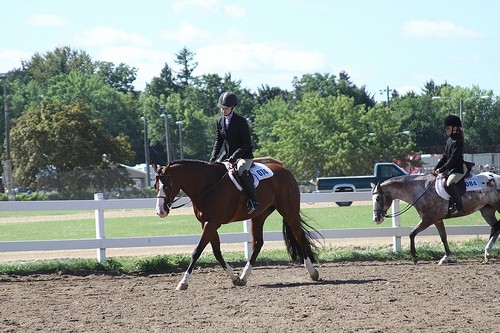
[
  {"x1": 446, "y1": 182, "x2": 462, "y2": 215},
  {"x1": 239, "y1": 175, "x2": 259, "y2": 214}
]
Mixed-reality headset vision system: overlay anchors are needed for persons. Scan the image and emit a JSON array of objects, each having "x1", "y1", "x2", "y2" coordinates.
[
  {"x1": 433, "y1": 115, "x2": 468, "y2": 216},
  {"x1": 209, "y1": 92, "x2": 260, "y2": 214}
]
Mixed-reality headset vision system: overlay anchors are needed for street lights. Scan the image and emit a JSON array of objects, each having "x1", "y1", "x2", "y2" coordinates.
[{"x1": 431, "y1": 95, "x2": 489, "y2": 129}]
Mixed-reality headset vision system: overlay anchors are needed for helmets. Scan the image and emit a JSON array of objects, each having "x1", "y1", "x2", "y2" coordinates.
[
  {"x1": 445, "y1": 115, "x2": 462, "y2": 127},
  {"x1": 217, "y1": 92, "x2": 237, "y2": 109}
]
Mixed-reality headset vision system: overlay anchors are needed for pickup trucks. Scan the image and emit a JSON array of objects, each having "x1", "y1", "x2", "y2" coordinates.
[{"x1": 315, "y1": 162, "x2": 409, "y2": 206}]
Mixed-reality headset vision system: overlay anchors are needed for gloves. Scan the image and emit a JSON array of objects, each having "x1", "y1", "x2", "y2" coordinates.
[{"x1": 228, "y1": 155, "x2": 237, "y2": 163}]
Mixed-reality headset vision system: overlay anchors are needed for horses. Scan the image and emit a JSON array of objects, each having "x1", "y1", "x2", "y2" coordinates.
[
  {"x1": 150, "y1": 158, "x2": 322, "y2": 292},
  {"x1": 369, "y1": 169, "x2": 500, "y2": 267}
]
